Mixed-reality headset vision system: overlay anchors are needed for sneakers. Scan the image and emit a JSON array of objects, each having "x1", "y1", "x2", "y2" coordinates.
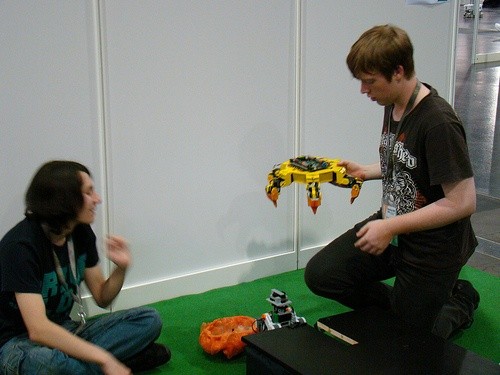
[{"x1": 121, "y1": 343, "x2": 171, "y2": 373}]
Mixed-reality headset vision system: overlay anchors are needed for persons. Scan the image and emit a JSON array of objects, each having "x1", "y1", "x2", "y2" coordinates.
[
  {"x1": 303, "y1": 25, "x2": 481, "y2": 339},
  {"x1": 0, "y1": 161, "x2": 171, "y2": 375}
]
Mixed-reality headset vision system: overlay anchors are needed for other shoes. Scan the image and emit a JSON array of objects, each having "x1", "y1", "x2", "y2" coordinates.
[{"x1": 452, "y1": 279, "x2": 479, "y2": 309}]
